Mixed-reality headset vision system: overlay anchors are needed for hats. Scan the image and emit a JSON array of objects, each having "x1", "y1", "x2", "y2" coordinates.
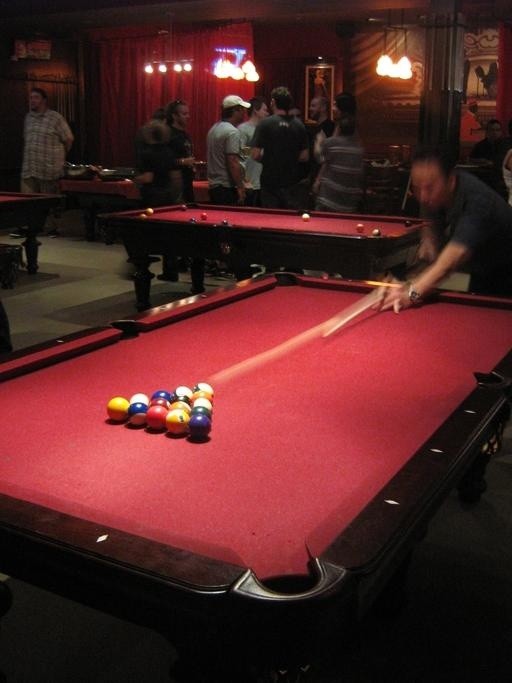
[{"x1": 222, "y1": 95, "x2": 251, "y2": 109}]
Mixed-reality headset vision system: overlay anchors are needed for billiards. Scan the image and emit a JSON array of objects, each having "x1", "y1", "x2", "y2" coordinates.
[
  {"x1": 147, "y1": 208, "x2": 153, "y2": 215},
  {"x1": 179, "y1": 203, "x2": 228, "y2": 225},
  {"x1": 321, "y1": 273, "x2": 328, "y2": 280},
  {"x1": 335, "y1": 274, "x2": 343, "y2": 279},
  {"x1": 107, "y1": 381, "x2": 216, "y2": 438},
  {"x1": 356, "y1": 224, "x2": 364, "y2": 232},
  {"x1": 139, "y1": 213, "x2": 147, "y2": 219},
  {"x1": 301, "y1": 213, "x2": 310, "y2": 222},
  {"x1": 373, "y1": 229, "x2": 380, "y2": 235},
  {"x1": 404, "y1": 220, "x2": 411, "y2": 226}
]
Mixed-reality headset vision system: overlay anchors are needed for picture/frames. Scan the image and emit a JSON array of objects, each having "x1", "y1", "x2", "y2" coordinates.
[{"x1": 304, "y1": 64, "x2": 335, "y2": 126}]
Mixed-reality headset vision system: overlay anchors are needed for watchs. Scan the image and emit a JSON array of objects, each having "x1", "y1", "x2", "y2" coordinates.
[{"x1": 409, "y1": 287, "x2": 419, "y2": 304}]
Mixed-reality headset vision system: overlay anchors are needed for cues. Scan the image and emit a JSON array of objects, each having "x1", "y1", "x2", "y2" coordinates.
[{"x1": 207, "y1": 288, "x2": 380, "y2": 385}]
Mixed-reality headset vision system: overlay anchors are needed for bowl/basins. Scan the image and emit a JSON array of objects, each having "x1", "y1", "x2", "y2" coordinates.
[{"x1": 62, "y1": 165, "x2": 91, "y2": 176}]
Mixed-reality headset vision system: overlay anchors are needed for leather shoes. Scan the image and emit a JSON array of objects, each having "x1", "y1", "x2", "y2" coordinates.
[{"x1": 157, "y1": 274, "x2": 177, "y2": 281}]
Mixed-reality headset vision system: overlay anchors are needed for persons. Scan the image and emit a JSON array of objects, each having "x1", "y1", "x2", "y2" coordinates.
[
  {"x1": 500, "y1": 139, "x2": 512, "y2": 205},
  {"x1": 7, "y1": 88, "x2": 75, "y2": 237},
  {"x1": 313, "y1": 70, "x2": 331, "y2": 98},
  {"x1": 466, "y1": 116, "x2": 508, "y2": 200},
  {"x1": 206, "y1": 85, "x2": 364, "y2": 278},
  {"x1": 373, "y1": 141, "x2": 512, "y2": 316},
  {"x1": 128, "y1": 101, "x2": 196, "y2": 280}
]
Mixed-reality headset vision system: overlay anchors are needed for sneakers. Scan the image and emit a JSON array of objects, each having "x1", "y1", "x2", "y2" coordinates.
[
  {"x1": 10, "y1": 229, "x2": 28, "y2": 236},
  {"x1": 48, "y1": 230, "x2": 56, "y2": 238}
]
